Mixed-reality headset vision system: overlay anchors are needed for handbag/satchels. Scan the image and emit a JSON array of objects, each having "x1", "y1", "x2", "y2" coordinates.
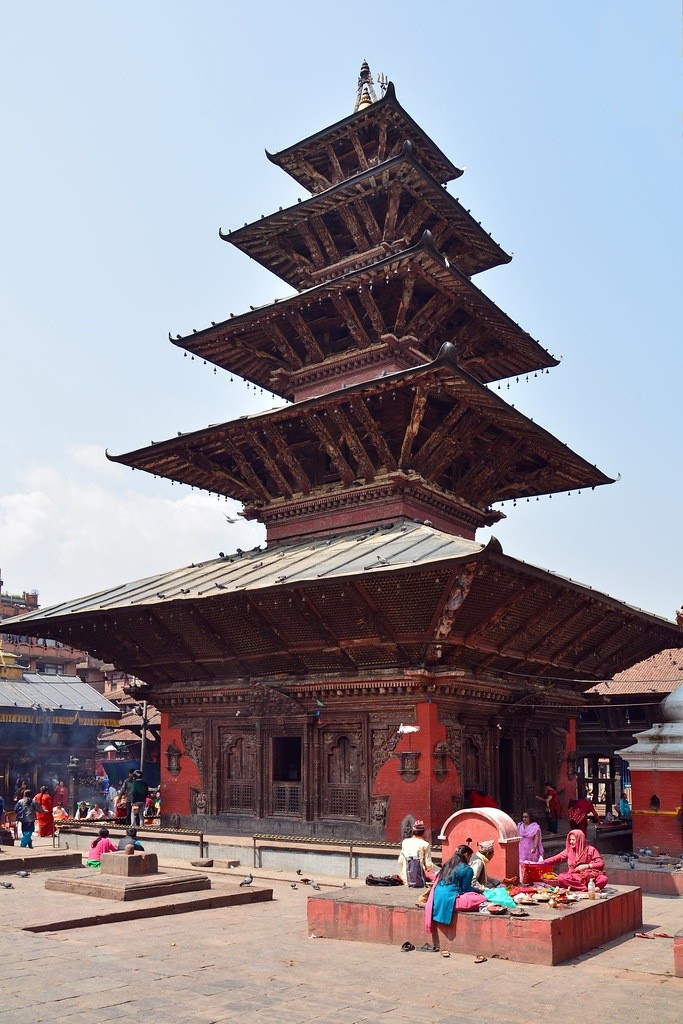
[
  {"x1": 16, "y1": 807, "x2": 24, "y2": 822},
  {"x1": 406, "y1": 855, "x2": 425, "y2": 888}
]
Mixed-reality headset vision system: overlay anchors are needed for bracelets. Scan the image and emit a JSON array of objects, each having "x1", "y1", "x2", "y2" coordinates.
[
  {"x1": 588, "y1": 864, "x2": 590, "y2": 869},
  {"x1": 534, "y1": 846, "x2": 536, "y2": 848}
]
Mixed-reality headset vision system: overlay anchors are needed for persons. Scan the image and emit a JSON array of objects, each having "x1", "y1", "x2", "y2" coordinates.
[
  {"x1": 397, "y1": 820, "x2": 442, "y2": 888},
  {"x1": 0, "y1": 796, "x2": 4, "y2": 845},
  {"x1": 32, "y1": 786, "x2": 69, "y2": 837},
  {"x1": 14, "y1": 773, "x2": 31, "y2": 801},
  {"x1": 116, "y1": 768, "x2": 148, "y2": 827},
  {"x1": 517, "y1": 810, "x2": 544, "y2": 884},
  {"x1": 535, "y1": 782, "x2": 564, "y2": 836},
  {"x1": 568, "y1": 798, "x2": 601, "y2": 839},
  {"x1": 14, "y1": 789, "x2": 48, "y2": 848},
  {"x1": 108, "y1": 779, "x2": 124, "y2": 811},
  {"x1": 144, "y1": 785, "x2": 160, "y2": 817},
  {"x1": 53, "y1": 780, "x2": 68, "y2": 807},
  {"x1": 87, "y1": 828, "x2": 144, "y2": 868},
  {"x1": 618, "y1": 793, "x2": 632, "y2": 829},
  {"x1": 75, "y1": 801, "x2": 116, "y2": 821},
  {"x1": 424, "y1": 840, "x2": 517, "y2": 934},
  {"x1": 541, "y1": 830, "x2": 609, "y2": 892}
]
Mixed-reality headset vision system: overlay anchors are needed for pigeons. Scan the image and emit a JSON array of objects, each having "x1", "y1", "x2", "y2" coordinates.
[
  {"x1": 620, "y1": 845, "x2": 682, "y2": 870},
  {"x1": 156, "y1": 544, "x2": 261, "y2": 598},
  {"x1": 0, "y1": 882, "x2": 15, "y2": 889},
  {"x1": 290, "y1": 868, "x2": 347, "y2": 891},
  {"x1": 239, "y1": 874, "x2": 254, "y2": 887},
  {"x1": 397, "y1": 722, "x2": 421, "y2": 734},
  {"x1": 223, "y1": 511, "x2": 243, "y2": 523},
  {"x1": 15, "y1": 871, "x2": 30, "y2": 877},
  {"x1": 317, "y1": 699, "x2": 326, "y2": 706},
  {"x1": 377, "y1": 555, "x2": 390, "y2": 565},
  {"x1": 357, "y1": 517, "x2": 434, "y2": 542}
]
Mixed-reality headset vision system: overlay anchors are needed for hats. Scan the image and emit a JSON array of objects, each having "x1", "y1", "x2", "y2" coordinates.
[{"x1": 480, "y1": 841, "x2": 494, "y2": 852}]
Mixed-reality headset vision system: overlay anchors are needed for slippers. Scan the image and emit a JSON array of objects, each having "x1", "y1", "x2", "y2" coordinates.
[
  {"x1": 654, "y1": 933, "x2": 674, "y2": 937},
  {"x1": 441, "y1": 949, "x2": 450, "y2": 957},
  {"x1": 474, "y1": 955, "x2": 487, "y2": 963},
  {"x1": 416, "y1": 942, "x2": 439, "y2": 952},
  {"x1": 634, "y1": 933, "x2": 656, "y2": 939},
  {"x1": 401, "y1": 942, "x2": 415, "y2": 952}
]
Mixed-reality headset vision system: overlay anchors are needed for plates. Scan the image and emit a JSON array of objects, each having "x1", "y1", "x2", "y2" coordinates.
[
  {"x1": 487, "y1": 906, "x2": 507, "y2": 914},
  {"x1": 532, "y1": 897, "x2": 550, "y2": 900},
  {"x1": 511, "y1": 911, "x2": 528, "y2": 916},
  {"x1": 521, "y1": 901, "x2": 537, "y2": 904}
]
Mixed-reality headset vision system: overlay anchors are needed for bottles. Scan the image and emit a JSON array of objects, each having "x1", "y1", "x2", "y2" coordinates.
[
  {"x1": 547, "y1": 896, "x2": 557, "y2": 908},
  {"x1": 588, "y1": 879, "x2": 595, "y2": 900}
]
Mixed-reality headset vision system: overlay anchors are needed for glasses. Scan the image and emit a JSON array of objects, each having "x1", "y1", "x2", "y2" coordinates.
[{"x1": 522, "y1": 816, "x2": 527, "y2": 818}]
